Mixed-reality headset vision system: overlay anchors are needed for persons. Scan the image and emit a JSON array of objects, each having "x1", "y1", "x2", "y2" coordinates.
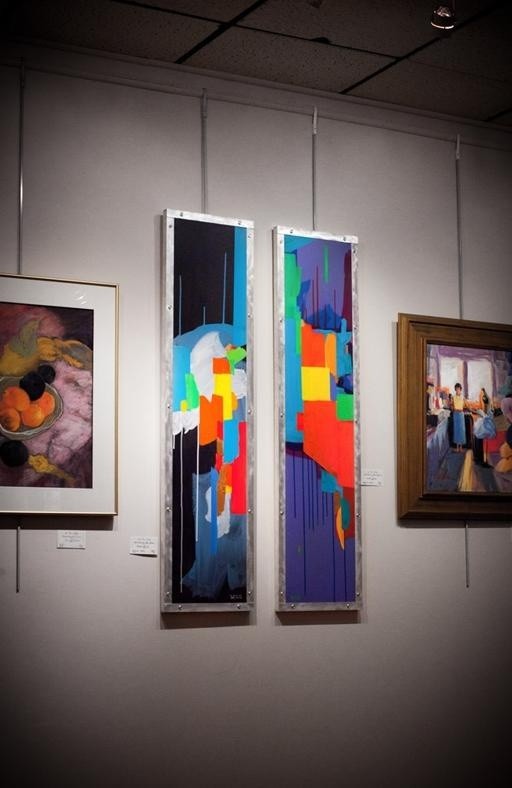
[
  {"x1": 425, "y1": 382, "x2": 439, "y2": 427},
  {"x1": 448, "y1": 383, "x2": 478, "y2": 453}
]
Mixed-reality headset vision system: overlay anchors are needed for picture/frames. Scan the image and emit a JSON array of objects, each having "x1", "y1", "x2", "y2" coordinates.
[
  {"x1": 160, "y1": 207, "x2": 256, "y2": 615},
  {"x1": 0, "y1": 272, "x2": 120, "y2": 515},
  {"x1": 395, "y1": 313, "x2": 512, "y2": 521},
  {"x1": 271, "y1": 223, "x2": 363, "y2": 613}
]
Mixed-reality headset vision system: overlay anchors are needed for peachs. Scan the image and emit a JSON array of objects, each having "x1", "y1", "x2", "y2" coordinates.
[{"x1": 0, "y1": 387, "x2": 54, "y2": 432}]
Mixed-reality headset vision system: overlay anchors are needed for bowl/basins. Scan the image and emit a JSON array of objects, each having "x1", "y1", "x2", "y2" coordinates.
[{"x1": 0, "y1": 375, "x2": 63, "y2": 441}]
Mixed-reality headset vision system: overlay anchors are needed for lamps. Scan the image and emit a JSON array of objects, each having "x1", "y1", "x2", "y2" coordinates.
[{"x1": 431, "y1": 0, "x2": 457, "y2": 30}]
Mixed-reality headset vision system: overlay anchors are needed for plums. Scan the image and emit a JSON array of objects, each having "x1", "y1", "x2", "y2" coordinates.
[
  {"x1": 0, "y1": 441, "x2": 29, "y2": 468},
  {"x1": 17, "y1": 363, "x2": 55, "y2": 401}
]
